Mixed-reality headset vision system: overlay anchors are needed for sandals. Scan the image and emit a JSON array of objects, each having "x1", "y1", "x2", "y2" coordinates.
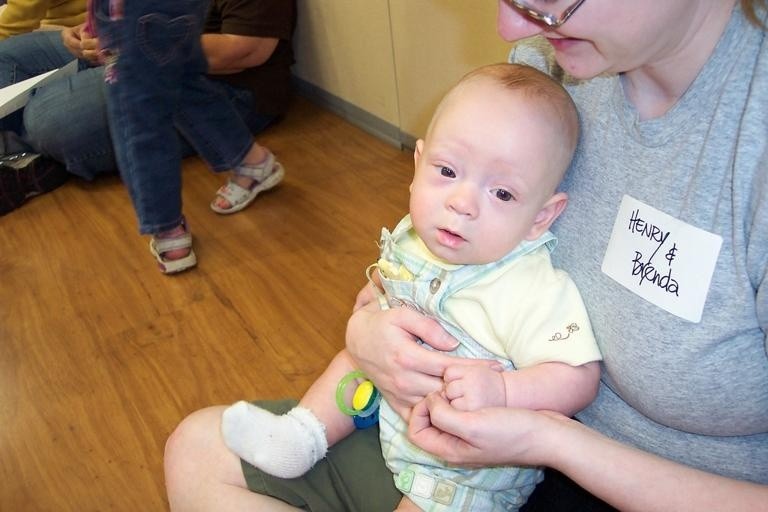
[
  {"x1": 148, "y1": 217, "x2": 201, "y2": 279},
  {"x1": 208, "y1": 148, "x2": 285, "y2": 213}
]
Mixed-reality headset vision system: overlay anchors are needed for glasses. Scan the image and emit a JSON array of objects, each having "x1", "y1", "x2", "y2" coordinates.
[{"x1": 502, "y1": 0, "x2": 588, "y2": 31}]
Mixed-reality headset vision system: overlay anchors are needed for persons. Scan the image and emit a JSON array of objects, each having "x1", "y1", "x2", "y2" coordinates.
[
  {"x1": 0, "y1": 0, "x2": 307, "y2": 180},
  {"x1": 225, "y1": 61, "x2": 599, "y2": 512},
  {"x1": 84, "y1": 0, "x2": 287, "y2": 276},
  {"x1": 166, "y1": 1, "x2": 768, "y2": 511},
  {"x1": 0, "y1": 0, "x2": 91, "y2": 36}
]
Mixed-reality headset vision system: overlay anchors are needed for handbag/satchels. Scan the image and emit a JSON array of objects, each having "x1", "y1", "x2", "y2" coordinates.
[{"x1": 0, "y1": 146, "x2": 70, "y2": 219}]
